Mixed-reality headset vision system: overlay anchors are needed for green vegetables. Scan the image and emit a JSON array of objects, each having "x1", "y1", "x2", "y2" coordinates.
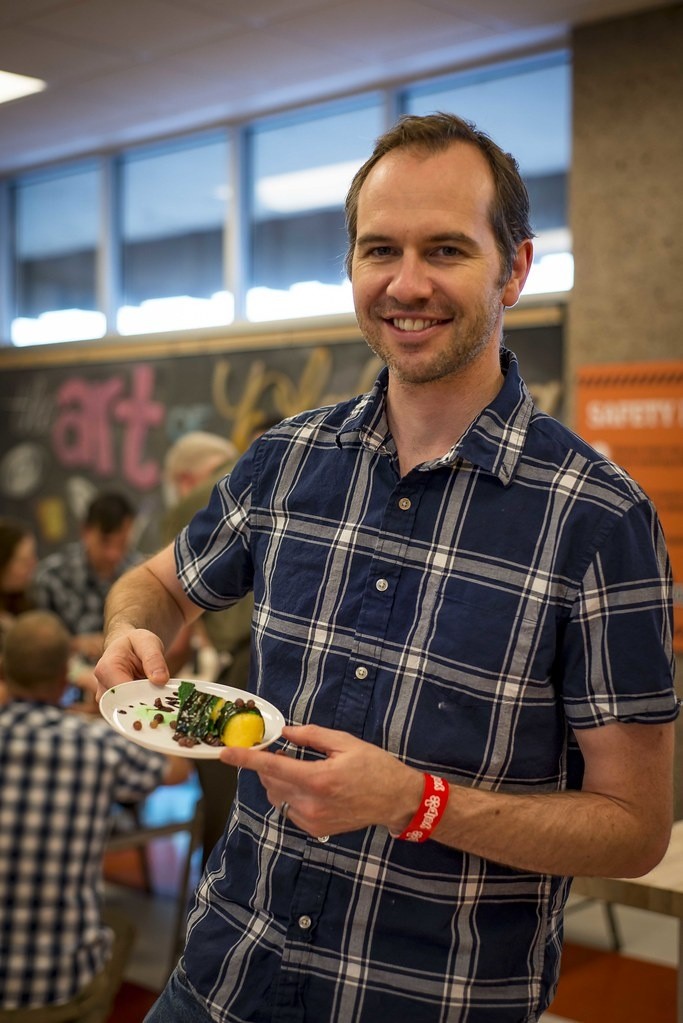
[{"x1": 177, "y1": 681, "x2": 264, "y2": 740}]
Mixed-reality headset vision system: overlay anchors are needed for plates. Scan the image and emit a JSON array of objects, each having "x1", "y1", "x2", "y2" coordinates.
[{"x1": 99, "y1": 678, "x2": 286, "y2": 759}]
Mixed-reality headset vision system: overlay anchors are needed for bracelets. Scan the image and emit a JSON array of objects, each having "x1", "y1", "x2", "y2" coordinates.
[
  {"x1": 280, "y1": 801, "x2": 290, "y2": 818},
  {"x1": 389, "y1": 772, "x2": 450, "y2": 843}
]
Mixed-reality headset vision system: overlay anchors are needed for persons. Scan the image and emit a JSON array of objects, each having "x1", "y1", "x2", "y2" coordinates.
[
  {"x1": 93, "y1": 110, "x2": 681, "y2": 1023},
  {"x1": 0, "y1": 488, "x2": 203, "y2": 1023}
]
